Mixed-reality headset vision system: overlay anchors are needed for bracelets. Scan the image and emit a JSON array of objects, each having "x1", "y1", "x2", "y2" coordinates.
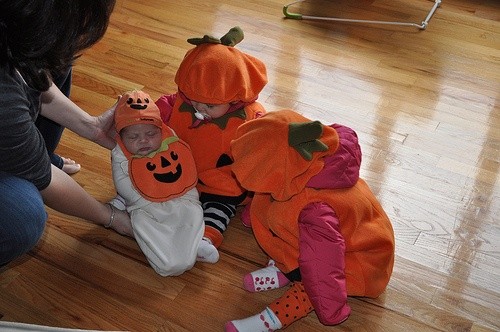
[{"x1": 106, "y1": 202, "x2": 115, "y2": 228}]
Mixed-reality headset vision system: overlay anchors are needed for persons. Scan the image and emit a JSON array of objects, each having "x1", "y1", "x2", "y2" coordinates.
[
  {"x1": 163, "y1": 25, "x2": 269, "y2": 263},
  {"x1": 216, "y1": 109, "x2": 396, "y2": 332},
  {"x1": 109, "y1": 89, "x2": 205, "y2": 276},
  {"x1": 0, "y1": 0, "x2": 116, "y2": 271}
]
